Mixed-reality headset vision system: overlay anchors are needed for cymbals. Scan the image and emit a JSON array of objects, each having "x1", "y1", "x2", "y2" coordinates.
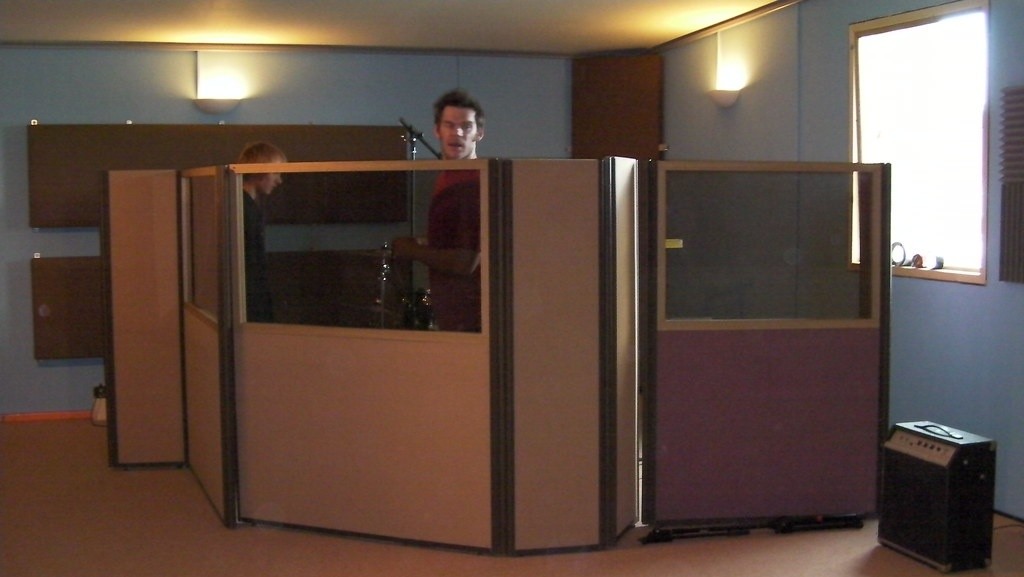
[{"x1": 356, "y1": 246, "x2": 410, "y2": 257}]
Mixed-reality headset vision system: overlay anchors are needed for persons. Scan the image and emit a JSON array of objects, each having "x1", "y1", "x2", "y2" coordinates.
[
  {"x1": 390, "y1": 88, "x2": 487, "y2": 332},
  {"x1": 235, "y1": 140, "x2": 301, "y2": 321}
]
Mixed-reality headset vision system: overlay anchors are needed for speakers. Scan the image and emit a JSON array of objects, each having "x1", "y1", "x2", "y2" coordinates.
[{"x1": 878, "y1": 419, "x2": 996, "y2": 573}]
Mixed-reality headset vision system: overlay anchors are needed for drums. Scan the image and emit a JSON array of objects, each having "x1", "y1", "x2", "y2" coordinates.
[{"x1": 405, "y1": 286, "x2": 441, "y2": 332}]
